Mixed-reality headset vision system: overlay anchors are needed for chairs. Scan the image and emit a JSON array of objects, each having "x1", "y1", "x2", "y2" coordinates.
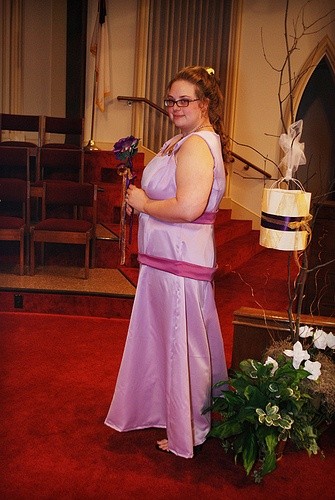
[
  {"x1": 27, "y1": 180, "x2": 99, "y2": 279},
  {"x1": 37, "y1": 113, "x2": 86, "y2": 184},
  {"x1": 0, "y1": 112, "x2": 43, "y2": 179},
  {"x1": 0, "y1": 146, "x2": 31, "y2": 276}
]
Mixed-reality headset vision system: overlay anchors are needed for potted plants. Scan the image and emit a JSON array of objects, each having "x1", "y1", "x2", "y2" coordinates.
[{"x1": 198, "y1": 357, "x2": 322, "y2": 482}]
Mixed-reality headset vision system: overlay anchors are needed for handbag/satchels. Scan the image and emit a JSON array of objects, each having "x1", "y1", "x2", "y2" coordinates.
[{"x1": 260, "y1": 177, "x2": 311, "y2": 251}]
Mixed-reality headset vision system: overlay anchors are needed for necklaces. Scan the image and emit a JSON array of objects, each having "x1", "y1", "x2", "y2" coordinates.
[{"x1": 164, "y1": 119, "x2": 207, "y2": 155}]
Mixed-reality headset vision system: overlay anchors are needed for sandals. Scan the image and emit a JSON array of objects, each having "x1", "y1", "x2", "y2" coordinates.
[{"x1": 156, "y1": 437, "x2": 202, "y2": 453}]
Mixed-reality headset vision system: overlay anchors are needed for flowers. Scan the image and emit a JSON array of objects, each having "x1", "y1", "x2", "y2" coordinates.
[{"x1": 113, "y1": 136, "x2": 141, "y2": 245}]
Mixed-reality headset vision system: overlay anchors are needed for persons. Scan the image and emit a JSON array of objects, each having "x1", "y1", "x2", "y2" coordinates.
[{"x1": 104, "y1": 65, "x2": 229, "y2": 457}]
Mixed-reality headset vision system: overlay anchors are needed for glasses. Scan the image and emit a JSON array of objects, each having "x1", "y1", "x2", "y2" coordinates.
[{"x1": 164, "y1": 99, "x2": 200, "y2": 107}]
